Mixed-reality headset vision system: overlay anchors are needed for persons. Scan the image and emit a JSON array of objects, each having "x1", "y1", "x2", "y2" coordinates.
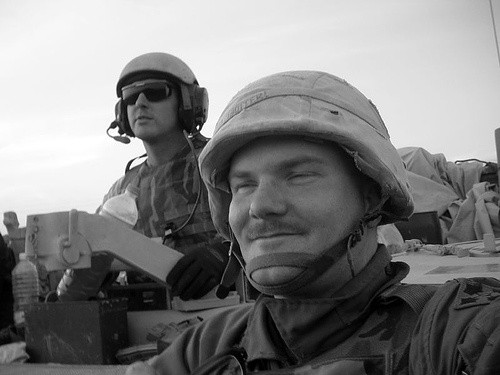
[
  {"x1": 120, "y1": 70, "x2": 500, "y2": 375},
  {"x1": 45, "y1": 52, "x2": 244, "y2": 306}
]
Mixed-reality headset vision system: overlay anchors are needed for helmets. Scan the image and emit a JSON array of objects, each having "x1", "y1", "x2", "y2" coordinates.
[
  {"x1": 117, "y1": 53, "x2": 199, "y2": 98},
  {"x1": 197, "y1": 70, "x2": 415, "y2": 245}
]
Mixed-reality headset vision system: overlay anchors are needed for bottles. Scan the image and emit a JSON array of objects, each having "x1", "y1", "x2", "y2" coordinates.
[
  {"x1": 55, "y1": 182, "x2": 142, "y2": 302},
  {"x1": 12, "y1": 252, "x2": 39, "y2": 327}
]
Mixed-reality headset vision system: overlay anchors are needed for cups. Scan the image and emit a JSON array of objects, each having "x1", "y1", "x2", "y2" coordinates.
[{"x1": 483, "y1": 233, "x2": 496, "y2": 252}]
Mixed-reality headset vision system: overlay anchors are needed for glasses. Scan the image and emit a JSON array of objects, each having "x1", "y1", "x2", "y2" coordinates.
[{"x1": 121, "y1": 82, "x2": 178, "y2": 105}]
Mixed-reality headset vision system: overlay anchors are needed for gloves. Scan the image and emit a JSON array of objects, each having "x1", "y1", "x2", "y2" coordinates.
[{"x1": 166, "y1": 236, "x2": 246, "y2": 301}]
